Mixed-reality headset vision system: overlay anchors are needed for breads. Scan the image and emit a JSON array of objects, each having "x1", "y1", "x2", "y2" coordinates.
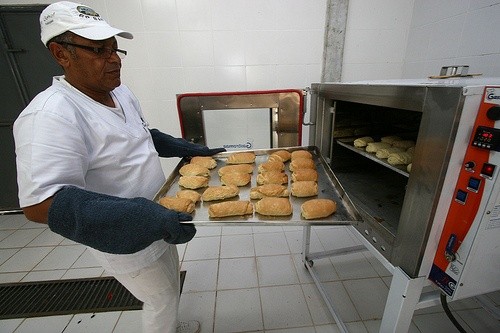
[
  {"x1": 159, "y1": 150, "x2": 335, "y2": 219},
  {"x1": 353, "y1": 136, "x2": 417, "y2": 172}
]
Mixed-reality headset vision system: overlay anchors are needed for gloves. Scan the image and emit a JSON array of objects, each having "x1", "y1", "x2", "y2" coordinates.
[
  {"x1": 48, "y1": 188, "x2": 196, "y2": 254},
  {"x1": 149, "y1": 128, "x2": 227, "y2": 159}
]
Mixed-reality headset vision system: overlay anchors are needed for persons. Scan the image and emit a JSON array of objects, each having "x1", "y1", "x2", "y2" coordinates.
[{"x1": 13, "y1": 0, "x2": 227, "y2": 333}]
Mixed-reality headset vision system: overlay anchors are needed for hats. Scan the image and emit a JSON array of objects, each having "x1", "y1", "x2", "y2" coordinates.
[{"x1": 39, "y1": 1, "x2": 133, "y2": 49}]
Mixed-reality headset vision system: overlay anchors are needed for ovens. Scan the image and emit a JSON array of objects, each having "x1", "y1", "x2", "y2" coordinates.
[{"x1": 175, "y1": 79, "x2": 500, "y2": 302}]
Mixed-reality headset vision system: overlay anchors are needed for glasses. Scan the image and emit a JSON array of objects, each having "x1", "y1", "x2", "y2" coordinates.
[{"x1": 57, "y1": 41, "x2": 127, "y2": 59}]
